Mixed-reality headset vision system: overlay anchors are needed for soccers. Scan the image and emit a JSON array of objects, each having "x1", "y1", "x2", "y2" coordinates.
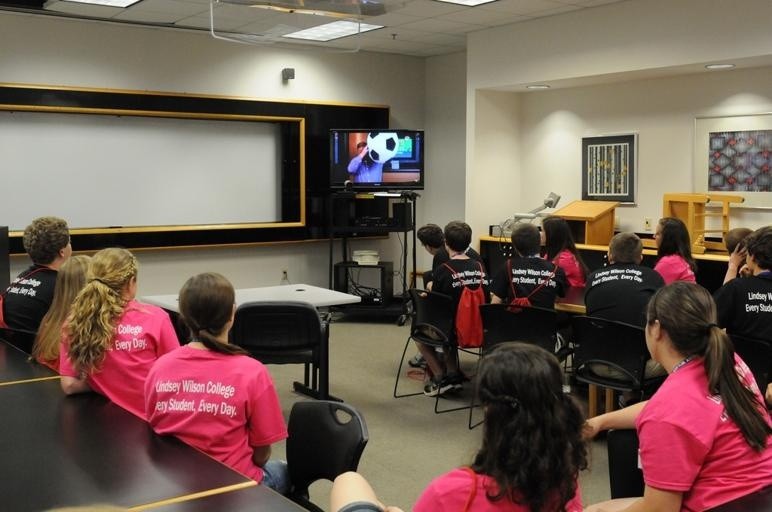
[{"x1": 366, "y1": 132, "x2": 399, "y2": 164}]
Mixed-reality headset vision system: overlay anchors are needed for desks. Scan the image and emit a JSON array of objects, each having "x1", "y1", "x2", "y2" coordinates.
[
  {"x1": 0, "y1": 341, "x2": 63, "y2": 385},
  {"x1": 0, "y1": 378, "x2": 251, "y2": 511},
  {"x1": 478, "y1": 235, "x2": 739, "y2": 305},
  {"x1": 142, "y1": 284, "x2": 361, "y2": 402},
  {"x1": 137, "y1": 484, "x2": 309, "y2": 511}
]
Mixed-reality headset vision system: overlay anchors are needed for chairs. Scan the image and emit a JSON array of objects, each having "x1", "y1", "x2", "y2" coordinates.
[
  {"x1": 468, "y1": 304, "x2": 576, "y2": 430},
  {"x1": 395, "y1": 289, "x2": 481, "y2": 415},
  {"x1": 229, "y1": 301, "x2": 345, "y2": 403},
  {"x1": 571, "y1": 314, "x2": 661, "y2": 434},
  {"x1": 607, "y1": 427, "x2": 644, "y2": 497},
  {"x1": 275, "y1": 398, "x2": 369, "y2": 502}
]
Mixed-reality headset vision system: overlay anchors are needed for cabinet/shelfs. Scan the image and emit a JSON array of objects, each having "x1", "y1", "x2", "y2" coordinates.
[{"x1": 329, "y1": 193, "x2": 419, "y2": 324}]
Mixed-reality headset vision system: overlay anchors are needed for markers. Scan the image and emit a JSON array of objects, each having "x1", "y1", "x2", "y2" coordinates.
[{"x1": 108, "y1": 227, "x2": 122, "y2": 228}]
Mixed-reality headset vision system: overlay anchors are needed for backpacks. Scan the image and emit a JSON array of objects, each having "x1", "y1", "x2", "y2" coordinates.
[
  {"x1": 442, "y1": 262, "x2": 487, "y2": 348},
  {"x1": 507, "y1": 258, "x2": 557, "y2": 308}
]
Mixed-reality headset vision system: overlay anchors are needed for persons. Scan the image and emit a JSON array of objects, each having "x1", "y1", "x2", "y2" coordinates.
[
  {"x1": 59, "y1": 247, "x2": 181, "y2": 421},
  {"x1": 0, "y1": 217, "x2": 73, "y2": 355},
  {"x1": 330, "y1": 341, "x2": 592, "y2": 512},
  {"x1": 580, "y1": 281, "x2": 772, "y2": 512},
  {"x1": 408, "y1": 216, "x2": 772, "y2": 415},
  {"x1": 31, "y1": 254, "x2": 92, "y2": 374},
  {"x1": 143, "y1": 272, "x2": 309, "y2": 502},
  {"x1": 347, "y1": 142, "x2": 383, "y2": 182}
]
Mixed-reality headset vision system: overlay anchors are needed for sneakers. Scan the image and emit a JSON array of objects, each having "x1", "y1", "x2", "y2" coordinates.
[
  {"x1": 424, "y1": 374, "x2": 464, "y2": 397},
  {"x1": 408, "y1": 353, "x2": 428, "y2": 368}
]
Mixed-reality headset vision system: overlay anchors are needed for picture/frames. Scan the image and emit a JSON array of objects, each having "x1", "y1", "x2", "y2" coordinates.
[
  {"x1": 692, "y1": 112, "x2": 772, "y2": 210},
  {"x1": 579, "y1": 133, "x2": 639, "y2": 206}
]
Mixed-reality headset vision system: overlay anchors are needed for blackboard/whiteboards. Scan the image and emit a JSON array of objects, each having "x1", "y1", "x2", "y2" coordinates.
[{"x1": 0, "y1": 108, "x2": 281, "y2": 232}]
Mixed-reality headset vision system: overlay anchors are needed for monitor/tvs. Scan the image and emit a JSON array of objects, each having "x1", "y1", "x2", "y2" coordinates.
[{"x1": 328, "y1": 128, "x2": 425, "y2": 191}]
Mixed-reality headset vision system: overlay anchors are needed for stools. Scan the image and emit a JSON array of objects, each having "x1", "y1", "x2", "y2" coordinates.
[{"x1": 662, "y1": 191, "x2": 745, "y2": 254}]
